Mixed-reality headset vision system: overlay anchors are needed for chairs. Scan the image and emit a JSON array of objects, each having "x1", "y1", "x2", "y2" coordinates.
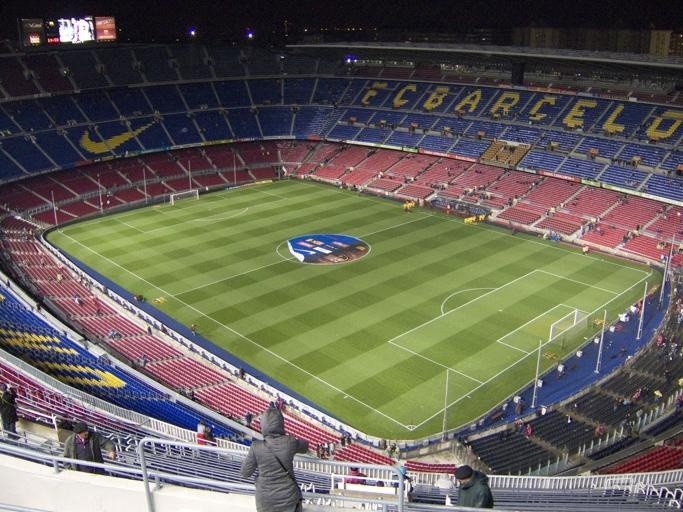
[
  {"x1": 632, "y1": 179, "x2": 682, "y2": 510},
  {"x1": 231, "y1": 382, "x2": 630, "y2": 508},
  {"x1": 1, "y1": 203, "x2": 108, "y2": 471},
  {"x1": 1, "y1": 40, "x2": 682, "y2": 203},
  {"x1": 109, "y1": 297, "x2": 229, "y2": 492},
  {"x1": 482, "y1": 203, "x2": 632, "y2": 255}
]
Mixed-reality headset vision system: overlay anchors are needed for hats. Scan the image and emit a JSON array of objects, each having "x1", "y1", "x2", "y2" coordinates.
[
  {"x1": 454, "y1": 465, "x2": 474, "y2": 478},
  {"x1": 71, "y1": 422, "x2": 88, "y2": 432}
]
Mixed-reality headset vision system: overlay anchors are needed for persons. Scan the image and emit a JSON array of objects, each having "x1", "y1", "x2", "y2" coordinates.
[
  {"x1": 579, "y1": 254, "x2": 683, "y2": 438},
  {"x1": 314, "y1": 432, "x2": 355, "y2": 458},
  {"x1": 239, "y1": 407, "x2": 307, "y2": 512},
  {"x1": 454, "y1": 465, "x2": 493, "y2": 507},
  {"x1": 299, "y1": 77, "x2": 682, "y2": 254},
  {"x1": 0, "y1": 380, "x2": 22, "y2": 444},
  {"x1": 64, "y1": 422, "x2": 106, "y2": 477},
  {"x1": 5, "y1": 224, "x2": 125, "y2": 350},
  {"x1": 508, "y1": 398, "x2": 541, "y2": 444}
]
